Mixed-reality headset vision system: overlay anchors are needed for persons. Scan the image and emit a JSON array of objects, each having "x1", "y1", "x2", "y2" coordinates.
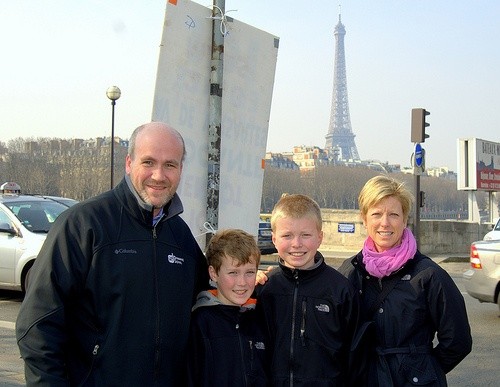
[
  {"x1": 15, "y1": 122, "x2": 269, "y2": 387},
  {"x1": 191, "y1": 229, "x2": 262, "y2": 386},
  {"x1": 254, "y1": 194, "x2": 370, "y2": 387},
  {"x1": 336, "y1": 176, "x2": 472, "y2": 387}
]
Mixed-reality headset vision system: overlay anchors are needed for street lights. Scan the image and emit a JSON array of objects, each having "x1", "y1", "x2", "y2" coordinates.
[{"x1": 105, "y1": 85, "x2": 122, "y2": 190}]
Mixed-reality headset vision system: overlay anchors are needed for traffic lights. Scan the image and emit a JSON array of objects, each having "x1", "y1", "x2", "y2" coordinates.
[
  {"x1": 410, "y1": 109, "x2": 431, "y2": 143},
  {"x1": 421, "y1": 190, "x2": 427, "y2": 208}
]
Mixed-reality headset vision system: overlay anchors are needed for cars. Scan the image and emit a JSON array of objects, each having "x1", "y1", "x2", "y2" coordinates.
[
  {"x1": 462, "y1": 216, "x2": 500, "y2": 316},
  {"x1": 0, "y1": 193, "x2": 82, "y2": 295}
]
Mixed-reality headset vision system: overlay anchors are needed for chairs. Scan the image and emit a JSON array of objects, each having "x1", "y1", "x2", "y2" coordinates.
[{"x1": 18, "y1": 207, "x2": 37, "y2": 229}]
